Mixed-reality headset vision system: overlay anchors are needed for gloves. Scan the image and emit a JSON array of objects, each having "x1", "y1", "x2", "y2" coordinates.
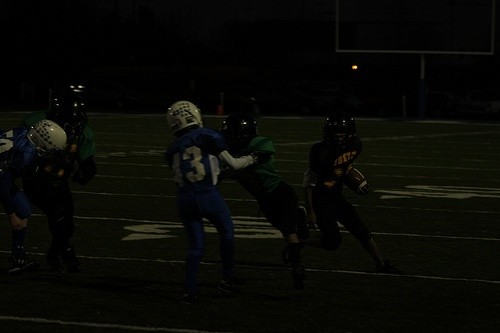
[
  {"x1": 250, "y1": 151, "x2": 272, "y2": 166},
  {"x1": 75, "y1": 157, "x2": 99, "y2": 185}
]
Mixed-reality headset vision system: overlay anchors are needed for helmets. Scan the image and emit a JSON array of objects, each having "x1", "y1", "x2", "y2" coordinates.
[
  {"x1": 52, "y1": 85, "x2": 88, "y2": 123},
  {"x1": 167, "y1": 101, "x2": 202, "y2": 130},
  {"x1": 323, "y1": 111, "x2": 356, "y2": 142},
  {"x1": 26, "y1": 120, "x2": 66, "y2": 151},
  {"x1": 222, "y1": 115, "x2": 256, "y2": 133}
]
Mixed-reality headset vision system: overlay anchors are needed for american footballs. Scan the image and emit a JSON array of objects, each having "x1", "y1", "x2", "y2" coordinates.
[{"x1": 343, "y1": 168, "x2": 369, "y2": 192}]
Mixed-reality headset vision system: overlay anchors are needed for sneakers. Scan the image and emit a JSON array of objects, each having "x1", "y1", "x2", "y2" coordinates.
[
  {"x1": 283, "y1": 239, "x2": 292, "y2": 263},
  {"x1": 376, "y1": 259, "x2": 402, "y2": 274}
]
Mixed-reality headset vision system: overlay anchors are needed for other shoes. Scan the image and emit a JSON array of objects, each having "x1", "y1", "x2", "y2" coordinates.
[
  {"x1": 292, "y1": 265, "x2": 306, "y2": 289},
  {"x1": 47, "y1": 254, "x2": 80, "y2": 274},
  {"x1": 186, "y1": 291, "x2": 199, "y2": 303},
  {"x1": 9, "y1": 256, "x2": 40, "y2": 272},
  {"x1": 225, "y1": 278, "x2": 247, "y2": 287}
]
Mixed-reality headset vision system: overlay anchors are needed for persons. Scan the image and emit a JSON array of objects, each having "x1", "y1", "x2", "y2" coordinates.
[
  {"x1": 302, "y1": 109, "x2": 403, "y2": 275},
  {"x1": 165, "y1": 100, "x2": 260, "y2": 303},
  {"x1": 0, "y1": 83, "x2": 97, "y2": 273},
  {"x1": 218, "y1": 110, "x2": 310, "y2": 291}
]
